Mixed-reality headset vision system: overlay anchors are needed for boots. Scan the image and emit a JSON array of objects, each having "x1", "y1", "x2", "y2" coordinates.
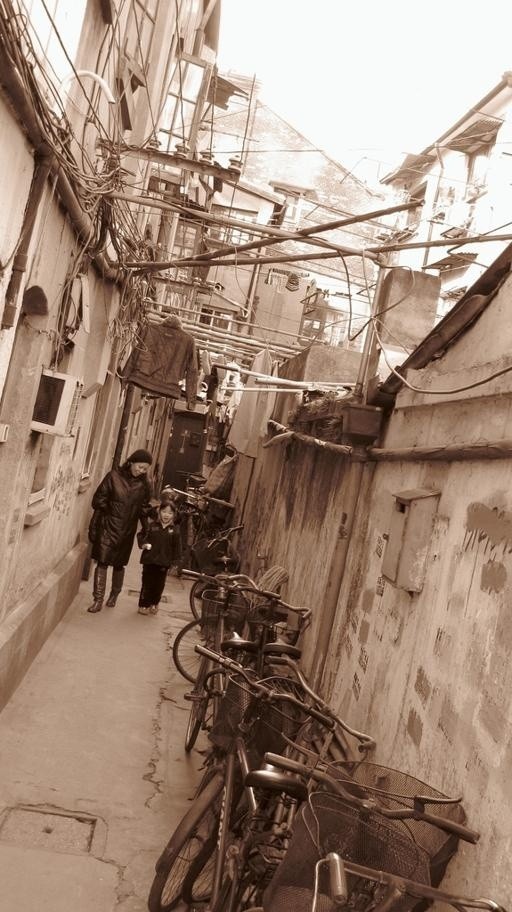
[
  {"x1": 87, "y1": 565, "x2": 107, "y2": 613},
  {"x1": 106, "y1": 567, "x2": 125, "y2": 607}
]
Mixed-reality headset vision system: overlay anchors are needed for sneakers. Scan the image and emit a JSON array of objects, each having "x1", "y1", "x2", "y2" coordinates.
[
  {"x1": 138, "y1": 607, "x2": 150, "y2": 614},
  {"x1": 150, "y1": 604, "x2": 159, "y2": 614}
]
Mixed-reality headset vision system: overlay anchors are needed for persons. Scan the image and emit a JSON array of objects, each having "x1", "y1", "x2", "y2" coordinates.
[
  {"x1": 137, "y1": 502, "x2": 182, "y2": 613},
  {"x1": 84, "y1": 451, "x2": 152, "y2": 613}
]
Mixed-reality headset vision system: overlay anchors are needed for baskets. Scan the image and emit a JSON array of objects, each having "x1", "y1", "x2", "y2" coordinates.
[
  {"x1": 322, "y1": 760, "x2": 466, "y2": 865},
  {"x1": 192, "y1": 536, "x2": 226, "y2": 575},
  {"x1": 207, "y1": 673, "x2": 312, "y2": 769},
  {"x1": 262, "y1": 790, "x2": 431, "y2": 912},
  {"x1": 201, "y1": 589, "x2": 248, "y2": 624},
  {"x1": 247, "y1": 603, "x2": 300, "y2": 646}
]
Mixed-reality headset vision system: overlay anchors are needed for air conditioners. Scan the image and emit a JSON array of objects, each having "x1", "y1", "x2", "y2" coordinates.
[{"x1": 30, "y1": 367, "x2": 83, "y2": 439}]
